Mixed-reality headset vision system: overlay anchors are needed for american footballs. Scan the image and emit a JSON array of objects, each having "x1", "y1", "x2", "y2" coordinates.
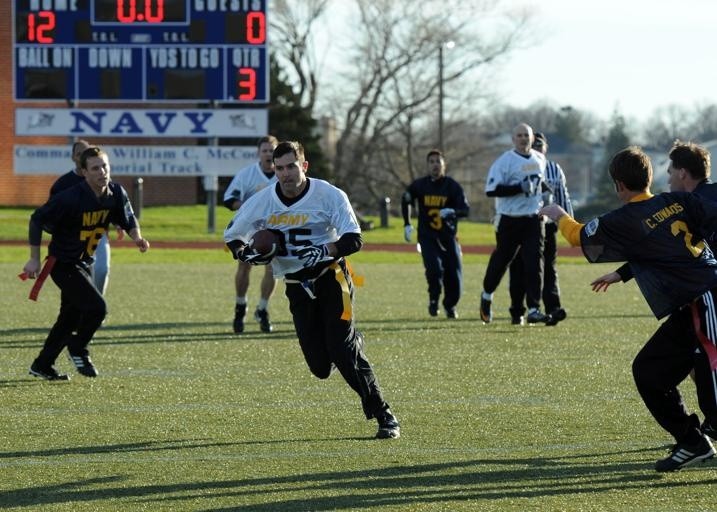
[{"x1": 248, "y1": 230, "x2": 276, "y2": 256}]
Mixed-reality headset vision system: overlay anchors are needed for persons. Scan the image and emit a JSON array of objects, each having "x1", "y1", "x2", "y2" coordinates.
[
  {"x1": 667, "y1": 144, "x2": 716, "y2": 383},
  {"x1": 536, "y1": 146, "x2": 717, "y2": 473},
  {"x1": 509, "y1": 131, "x2": 574, "y2": 327},
  {"x1": 401, "y1": 149, "x2": 470, "y2": 319},
  {"x1": 222, "y1": 134, "x2": 280, "y2": 334},
  {"x1": 23, "y1": 146, "x2": 150, "y2": 381},
  {"x1": 223, "y1": 141, "x2": 402, "y2": 440},
  {"x1": 48, "y1": 138, "x2": 126, "y2": 329},
  {"x1": 479, "y1": 121, "x2": 554, "y2": 324}
]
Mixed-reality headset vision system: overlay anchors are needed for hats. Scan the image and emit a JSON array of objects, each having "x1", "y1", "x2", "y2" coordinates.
[{"x1": 533, "y1": 133, "x2": 546, "y2": 147}]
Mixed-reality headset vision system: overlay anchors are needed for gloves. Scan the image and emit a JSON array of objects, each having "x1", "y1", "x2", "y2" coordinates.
[
  {"x1": 404, "y1": 225, "x2": 413, "y2": 242},
  {"x1": 440, "y1": 208, "x2": 455, "y2": 218},
  {"x1": 296, "y1": 244, "x2": 329, "y2": 268},
  {"x1": 235, "y1": 238, "x2": 277, "y2": 265},
  {"x1": 520, "y1": 175, "x2": 533, "y2": 193}
]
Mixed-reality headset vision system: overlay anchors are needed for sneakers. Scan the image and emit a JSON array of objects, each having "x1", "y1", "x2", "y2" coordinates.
[
  {"x1": 234, "y1": 304, "x2": 245, "y2": 332},
  {"x1": 355, "y1": 331, "x2": 362, "y2": 350},
  {"x1": 481, "y1": 292, "x2": 491, "y2": 322},
  {"x1": 376, "y1": 424, "x2": 401, "y2": 440},
  {"x1": 446, "y1": 305, "x2": 458, "y2": 318},
  {"x1": 255, "y1": 306, "x2": 270, "y2": 332},
  {"x1": 700, "y1": 420, "x2": 716, "y2": 440},
  {"x1": 513, "y1": 309, "x2": 567, "y2": 325},
  {"x1": 29, "y1": 359, "x2": 67, "y2": 380},
  {"x1": 430, "y1": 299, "x2": 438, "y2": 315},
  {"x1": 67, "y1": 347, "x2": 98, "y2": 377},
  {"x1": 655, "y1": 434, "x2": 716, "y2": 472}
]
